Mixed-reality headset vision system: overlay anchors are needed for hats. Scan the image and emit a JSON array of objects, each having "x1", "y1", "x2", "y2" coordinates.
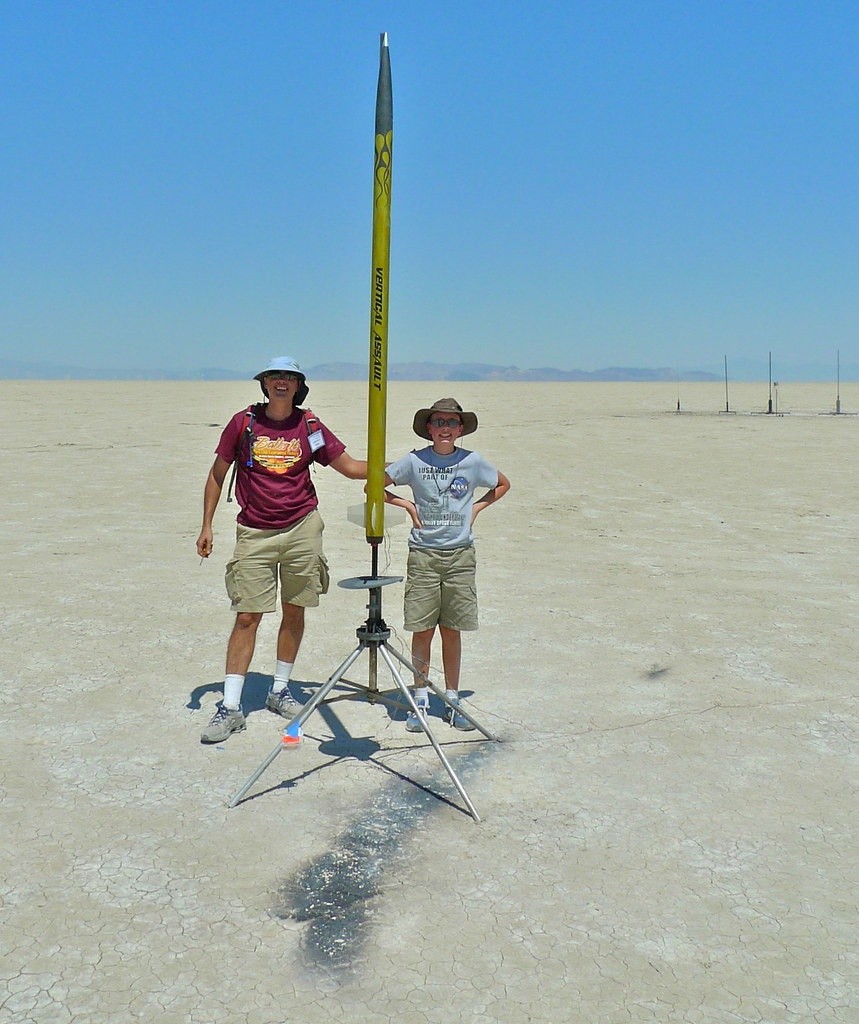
[
  {"x1": 253, "y1": 356, "x2": 306, "y2": 381},
  {"x1": 413, "y1": 398, "x2": 478, "y2": 441}
]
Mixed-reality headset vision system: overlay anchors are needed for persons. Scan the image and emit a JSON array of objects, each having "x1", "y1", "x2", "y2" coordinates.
[
  {"x1": 195, "y1": 355, "x2": 417, "y2": 745},
  {"x1": 364, "y1": 398, "x2": 510, "y2": 733}
]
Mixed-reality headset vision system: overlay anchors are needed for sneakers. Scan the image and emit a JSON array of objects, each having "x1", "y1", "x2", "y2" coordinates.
[
  {"x1": 406, "y1": 696, "x2": 430, "y2": 732},
  {"x1": 266, "y1": 684, "x2": 305, "y2": 719},
  {"x1": 443, "y1": 698, "x2": 476, "y2": 730},
  {"x1": 201, "y1": 704, "x2": 247, "y2": 742}
]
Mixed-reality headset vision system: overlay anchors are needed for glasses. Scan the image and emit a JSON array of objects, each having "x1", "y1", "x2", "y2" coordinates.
[
  {"x1": 430, "y1": 419, "x2": 461, "y2": 428},
  {"x1": 267, "y1": 372, "x2": 297, "y2": 381}
]
